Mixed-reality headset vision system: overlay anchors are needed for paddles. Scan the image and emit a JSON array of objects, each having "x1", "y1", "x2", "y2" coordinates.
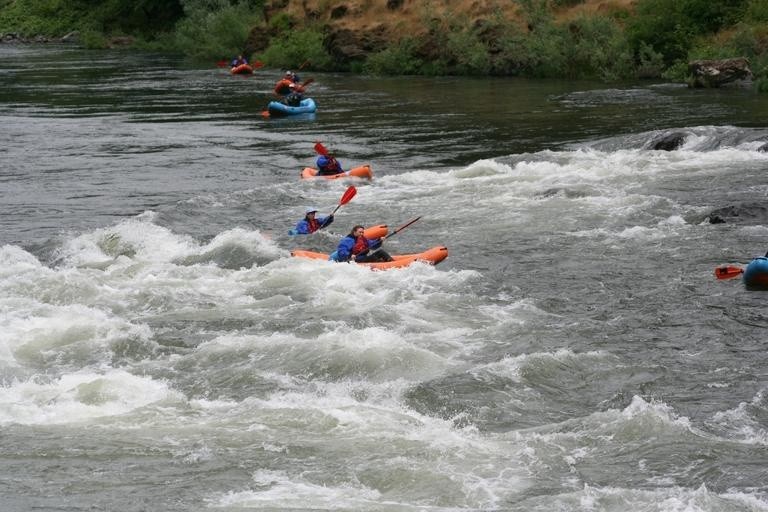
[
  {"x1": 314, "y1": 142, "x2": 345, "y2": 171},
  {"x1": 261, "y1": 77, "x2": 315, "y2": 116},
  {"x1": 715, "y1": 266, "x2": 746, "y2": 280},
  {"x1": 215, "y1": 61, "x2": 263, "y2": 69},
  {"x1": 345, "y1": 215, "x2": 420, "y2": 262},
  {"x1": 318, "y1": 185, "x2": 356, "y2": 229},
  {"x1": 288, "y1": 58, "x2": 309, "y2": 79}
]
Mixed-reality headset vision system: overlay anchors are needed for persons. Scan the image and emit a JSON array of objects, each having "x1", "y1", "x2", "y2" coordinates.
[
  {"x1": 312, "y1": 148, "x2": 342, "y2": 177},
  {"x1": 283, "y1": 69, "x2": 300, "y2": 84},
  {"x1": 231, "y1": 53, "x2": 248, "y2": 66},
  {"x1": 296, "y1": 207, "x2": 334, "y2": 233},
  {"x1": 337, "y1": 224, "x2": 397, "y2": 262},
  {"x1": 282, "y1": 83, "x2": 303, "y2": 107}
]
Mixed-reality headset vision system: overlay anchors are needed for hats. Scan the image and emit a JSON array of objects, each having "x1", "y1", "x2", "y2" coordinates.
[
  {"x1": 327, "y1": 151, "x2": 334, "y2": 155},
  {"x1": 306, "y1": 207, "x2": 318, "y2": 214},
  {"x1": 287, "y1": 71, "x2": 291, "y2": 75},
  {"x1": 288, "y1": 83, "x2": 296, "y2": 88}
]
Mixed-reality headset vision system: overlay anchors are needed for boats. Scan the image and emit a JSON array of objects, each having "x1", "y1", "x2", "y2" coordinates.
[
  {"x1": 268, "y1": 98, "x2": 317, "y2": 116},
  {"x1": 286, "y1": 223, "x2": 388, "y2": 242},
  {"x1": 744, "y1": 256, "x2": 768, "y2": 289},
  {"x1": 231, "y1": 64, "x2": 253, "y2": 75},
  {"x1": 299, "y1": 164, "x2": 372, "y2": 182},
  {"x1": 288, "y1": 244, "x2": 451, "y2": 272}
]
